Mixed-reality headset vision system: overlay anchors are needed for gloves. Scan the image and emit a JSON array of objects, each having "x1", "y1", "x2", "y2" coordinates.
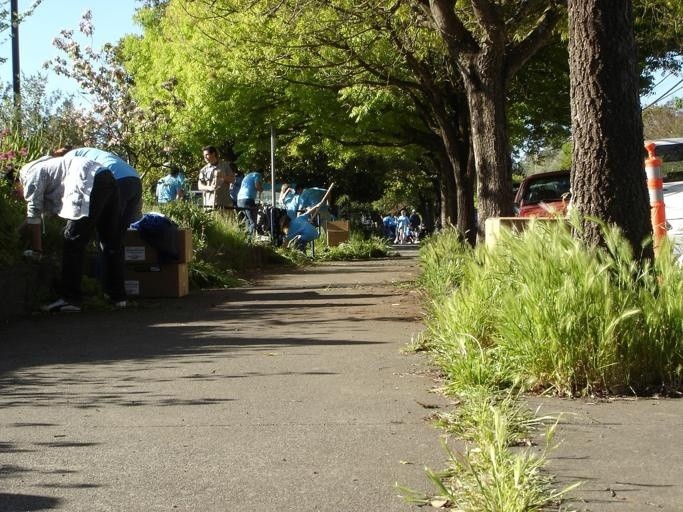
[{"x1": 24, "y1": 250, "x2": 41, "y2": 263}]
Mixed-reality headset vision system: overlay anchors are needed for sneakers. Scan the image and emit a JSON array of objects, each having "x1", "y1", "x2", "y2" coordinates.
[
  {"x1": 103, "y1": 292, "x2": 128, "y2": 311},
  {"x1": 40, "y1": 298, "x2": 81, "y2": 313}
]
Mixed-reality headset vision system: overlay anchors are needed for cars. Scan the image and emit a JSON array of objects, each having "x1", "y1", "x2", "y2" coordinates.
[
  {"x1": 510, "y1": 167, "x2": 573, "y2": 220},
  {"x1": 644, "y1": 138, "x2": 683, "y2": 241}
]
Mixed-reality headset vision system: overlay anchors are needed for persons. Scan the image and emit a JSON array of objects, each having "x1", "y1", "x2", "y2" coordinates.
[
  {"x1": 53, "y1": 145, "x2": 141, "y2": 235},
  {"x1": 383, "y1": 209, "x2": 422, "y2": 244},
  {"x1": 155, "y1": 144, "x2": 337, "y2": 262},
  {"x1": 5, "y1": 155, "x2": 126, "y2": 314}
]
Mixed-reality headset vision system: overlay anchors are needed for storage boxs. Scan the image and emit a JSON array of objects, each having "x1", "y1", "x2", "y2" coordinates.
[
  {"x1": 122, "y1": 261, "x2": 190, "y2": 297},
  {"x1": 127, "y1": 228, "x2": 193, "y2": 263},
  {"x1": 325, "y1": 220, "x2": 349, "y2": 246}
]
